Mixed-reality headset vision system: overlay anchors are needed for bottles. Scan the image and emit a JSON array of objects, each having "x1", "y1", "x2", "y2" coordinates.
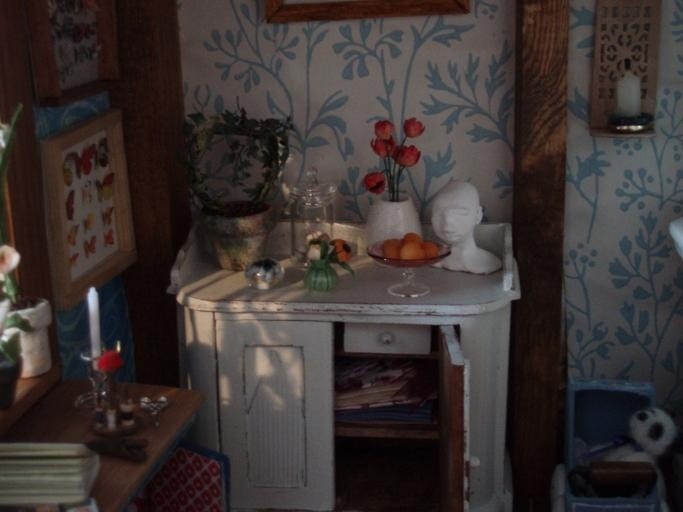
[{"x1": 291, "y1": 167, "x2": 334, "y2": 269}]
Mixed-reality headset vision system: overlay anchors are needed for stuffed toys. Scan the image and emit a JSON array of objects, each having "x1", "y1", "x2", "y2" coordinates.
[{"x1": 579, "y1": 406, "x2": 676, "y2": 466}]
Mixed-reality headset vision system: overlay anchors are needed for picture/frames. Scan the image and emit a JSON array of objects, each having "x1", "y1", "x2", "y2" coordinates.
[
  {"x1": 20, "y1": 0, "x2": 122, "y2": 98},
  {"x1": 37, "y1": 104, "x2": 139, "y2": 313},
  {"x1": 263, "y1": 0, "x2": 469, "y2": 25}
]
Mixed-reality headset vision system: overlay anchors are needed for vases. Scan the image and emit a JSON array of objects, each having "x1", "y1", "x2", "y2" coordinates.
[
  {"x1": 364, "y1": 199, "x2": 425, "y2": 258},
  {"x1": 1, "y1": 295, "x2": 55, "y2": 381}
]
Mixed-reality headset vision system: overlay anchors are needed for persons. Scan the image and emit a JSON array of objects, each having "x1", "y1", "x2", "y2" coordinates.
[{"x1": 429, "y1": 179, "x2": 503, "y2": 276}]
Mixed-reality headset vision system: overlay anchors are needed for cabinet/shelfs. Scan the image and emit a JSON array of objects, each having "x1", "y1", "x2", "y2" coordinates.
[{"x1": 163, "y1": 219, "x2": 529, "y2": 512}]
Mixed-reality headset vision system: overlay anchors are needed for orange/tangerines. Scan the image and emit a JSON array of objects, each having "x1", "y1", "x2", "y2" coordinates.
[
  {"x1": 331, "y1": 237, "x2": 351, "y2": 260},
  {"x1": 382, "y1": 232, "x2": 438, "y2": 260}
]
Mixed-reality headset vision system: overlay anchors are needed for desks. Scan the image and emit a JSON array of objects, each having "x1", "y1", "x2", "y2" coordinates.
[{"x1": 1, "y1": 380, "x2": 234, "y2": 511}]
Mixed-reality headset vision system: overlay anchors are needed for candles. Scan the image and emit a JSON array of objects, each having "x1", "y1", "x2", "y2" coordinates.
[
  {"x1": 84, "y1": 282, "x2": 104, "y2": 372},
  {"x1": 611, "y1": 54, "x2": 644, "y2": 120}
]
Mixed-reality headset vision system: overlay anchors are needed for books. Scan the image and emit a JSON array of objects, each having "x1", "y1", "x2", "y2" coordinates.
[{"x1": 0, "y1": 438, "x2": 99, "y2": 507}]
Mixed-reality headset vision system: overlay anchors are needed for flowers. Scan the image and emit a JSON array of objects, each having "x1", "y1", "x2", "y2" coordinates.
[
  {"x1": 363, "y1": 110, "x2": 427, "y2": 201},
  {"x1": 0, "y1": 242, "x2": 27, "y2": 306}
]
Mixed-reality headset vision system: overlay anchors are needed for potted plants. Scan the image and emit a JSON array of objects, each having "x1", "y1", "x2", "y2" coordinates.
[
  {"x1": 169, "y1": 105, "x2": 298, "y2": 279},
  {"x1": 0, "y1": 299, "x2": 36, "y2": 414}
]
